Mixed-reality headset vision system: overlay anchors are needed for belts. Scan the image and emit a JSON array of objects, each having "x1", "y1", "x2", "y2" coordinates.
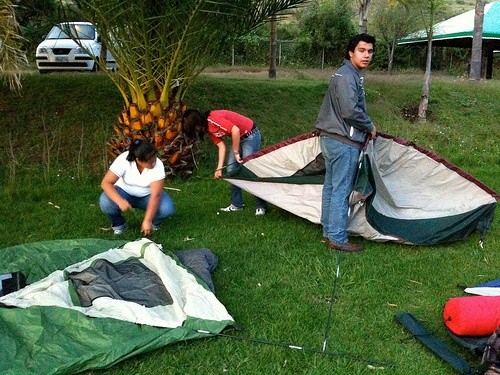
[{"x1": 240, "y1": 126, "x2": 259, "y2": 140}]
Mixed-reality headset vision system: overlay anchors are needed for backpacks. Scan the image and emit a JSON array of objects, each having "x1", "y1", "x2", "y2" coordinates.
[{"x1": 474, "y1": 329, "x2": 500, "y2": 375}]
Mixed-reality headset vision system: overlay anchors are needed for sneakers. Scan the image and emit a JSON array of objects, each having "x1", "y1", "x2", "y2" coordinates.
[
  {"x1": 255, "y1": 208, "x2": 265, "y2": 215},
  {"x1": 220, "y1": 204, "x2": 243, "y2": 212}
]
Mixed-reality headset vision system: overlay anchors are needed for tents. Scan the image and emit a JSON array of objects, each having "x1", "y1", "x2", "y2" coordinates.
[
  {"x1": 194, "y1": 132, "x2": 500, "y2": 247},
  {"x1": 1, "y1": 235, "x2": 243, "y2": 375}
]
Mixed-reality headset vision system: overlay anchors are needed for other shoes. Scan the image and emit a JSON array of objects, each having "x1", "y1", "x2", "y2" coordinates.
[
  {"x1": 114, "y1": 224, "x2": 129, "y2": 235},
  {"x1": 321, "y1": 236, "x2": 363, "y2": 251},
  {"x1": 152, "y1": 224, "x2": 160, "y2": 231}
]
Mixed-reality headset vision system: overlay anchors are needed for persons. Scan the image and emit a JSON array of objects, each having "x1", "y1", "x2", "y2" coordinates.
[
  {"x1": 181, "y1": 108, "x2": 267, "y2": 216},
  {"x1": 315, "y1": 34, "x2": 376, "y2": 252},
  {"x1": 99, "y1": 139, "x2": 174, "y2": 235}
]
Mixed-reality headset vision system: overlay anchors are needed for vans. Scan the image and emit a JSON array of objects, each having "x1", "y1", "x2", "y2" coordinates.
[{"x1": 36, "y1": 21, "x2": 122, "y2": 73}]
